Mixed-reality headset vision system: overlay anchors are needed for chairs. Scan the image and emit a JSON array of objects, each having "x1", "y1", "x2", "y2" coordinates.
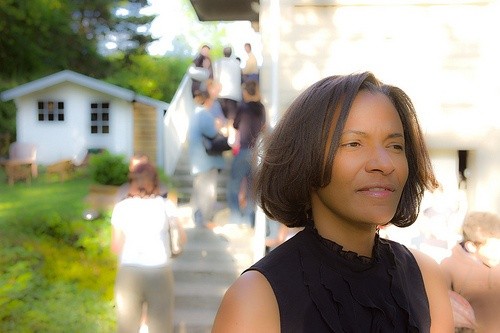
[{"x1": 8, "y1": 142, "x2": 38, "y2": 186}]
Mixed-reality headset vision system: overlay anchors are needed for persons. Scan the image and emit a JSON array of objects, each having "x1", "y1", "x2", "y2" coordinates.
[
  {"x1": 188, "y1": 43, "x2": 266, "y2": 227},
  {"x1": 441, "y1": 211, "x2": 500, "y2": 333},
  {"x1": 211, "y1": 72, "x2": 455, "y2": 333},
  {"x1": 110, "y1": 156, "x2": 186, "y2": 333}
]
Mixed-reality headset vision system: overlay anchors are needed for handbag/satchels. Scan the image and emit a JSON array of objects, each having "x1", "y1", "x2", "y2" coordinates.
[{"x1": 202, "y1": 131, "x2": 232, "y2": 156}]
